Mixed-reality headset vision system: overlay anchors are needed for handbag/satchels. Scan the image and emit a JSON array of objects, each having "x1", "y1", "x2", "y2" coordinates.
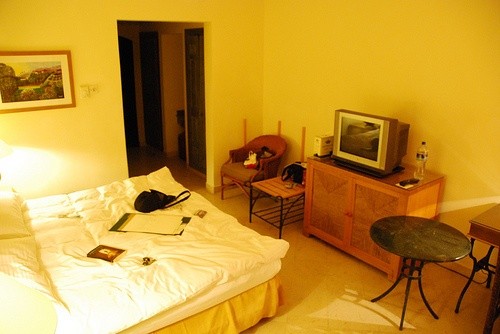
[{"x1": 134, "y1": 188, "x2": 190, "y2": 213}]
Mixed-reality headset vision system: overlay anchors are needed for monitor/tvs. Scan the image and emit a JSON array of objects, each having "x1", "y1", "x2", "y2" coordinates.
[{"x1": 331, "y1": 109, "x2": 409, "y2": 175}]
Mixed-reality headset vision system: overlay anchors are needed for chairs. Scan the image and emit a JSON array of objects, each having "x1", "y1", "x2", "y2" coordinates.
[{"x1": 220, "y1": 134, "x2": 287, "y2": 200}]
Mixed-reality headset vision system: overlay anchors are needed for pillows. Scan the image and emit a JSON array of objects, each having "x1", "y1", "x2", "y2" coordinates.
[
  {"x1": 0, "y1": 191, "x2": 31, "y2": 239},
  {"x1": 0, "y1": 235, "x2": 74, "y2": 334}
]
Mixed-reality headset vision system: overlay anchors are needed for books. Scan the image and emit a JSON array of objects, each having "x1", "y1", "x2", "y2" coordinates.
[{"x1": 87, "y1": 245, "x2": 127, "y2": 262}]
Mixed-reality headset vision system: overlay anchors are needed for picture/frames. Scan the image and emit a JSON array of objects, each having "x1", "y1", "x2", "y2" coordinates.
[{"x1": 0, "y1": 49, "x2": 77, "y2": 114}]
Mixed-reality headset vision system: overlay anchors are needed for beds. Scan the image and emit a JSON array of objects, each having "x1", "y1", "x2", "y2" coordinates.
[{"x1": 0, "y1": 166, "x2": 290, "y2": 334}]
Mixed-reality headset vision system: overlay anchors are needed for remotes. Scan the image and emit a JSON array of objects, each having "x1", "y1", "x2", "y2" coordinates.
[{"x1": 400, "y1": 179, "x2": 419, "y2": 187}]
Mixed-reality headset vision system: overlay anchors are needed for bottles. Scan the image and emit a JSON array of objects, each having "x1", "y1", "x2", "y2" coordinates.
[{"x1": 414, "y1": 141, "x2": 428, "y2": 179}]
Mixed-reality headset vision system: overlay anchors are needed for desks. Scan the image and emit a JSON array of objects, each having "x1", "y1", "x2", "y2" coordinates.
[
  {"x1": 455, "y1": 202, "x2": 500, "y2": 313},
  {"x1": 369, "y1": 215, "x2": 472, "y2": 331},
  {"x1": 249, "y1": 176, "x2": 305, "y2": 239}
]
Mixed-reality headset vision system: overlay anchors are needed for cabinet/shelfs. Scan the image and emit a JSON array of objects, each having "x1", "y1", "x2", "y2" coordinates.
[{"x1": 302, "y1": 156, "x2": 445, "y2": 283}]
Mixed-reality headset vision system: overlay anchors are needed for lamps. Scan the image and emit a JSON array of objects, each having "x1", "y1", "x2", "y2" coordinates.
[{"x1": 0, "y1": 271, "x2": 58, "y2": 334}]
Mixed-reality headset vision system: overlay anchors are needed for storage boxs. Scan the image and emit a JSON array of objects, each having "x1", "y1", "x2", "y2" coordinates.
[{"x1": 314, "y1": 134, "x2": 334, "y2": 156}]
